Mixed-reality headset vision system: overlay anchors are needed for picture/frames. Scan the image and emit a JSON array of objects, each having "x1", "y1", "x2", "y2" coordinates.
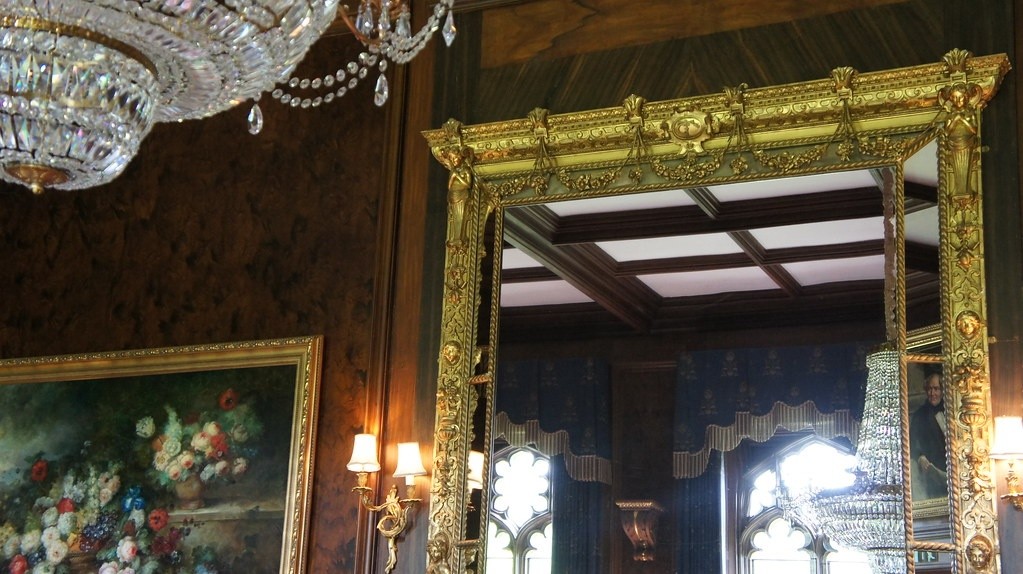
[{"x1": 0, "y1": 332, "x2": 325, "y2": 574}]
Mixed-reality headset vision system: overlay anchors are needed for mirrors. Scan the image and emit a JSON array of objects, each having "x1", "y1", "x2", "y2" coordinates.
[{"x1": 418, "y1": 48, "x2": 1011, "y2": 574}]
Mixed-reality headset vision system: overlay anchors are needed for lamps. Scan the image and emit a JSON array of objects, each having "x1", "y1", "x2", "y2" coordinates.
[
  {"x1": 345, "y1": 433, "x2": 428, "y2": 574},
  {"x1": 0, "y1": 0, "x2": 461, "y2": 195},
  {"x1": 612, "y1": 496, "x2": 667, "y2": 561},
  {"x1": 810, "y1": 165, "x2": 915, "y2": 574}
]
